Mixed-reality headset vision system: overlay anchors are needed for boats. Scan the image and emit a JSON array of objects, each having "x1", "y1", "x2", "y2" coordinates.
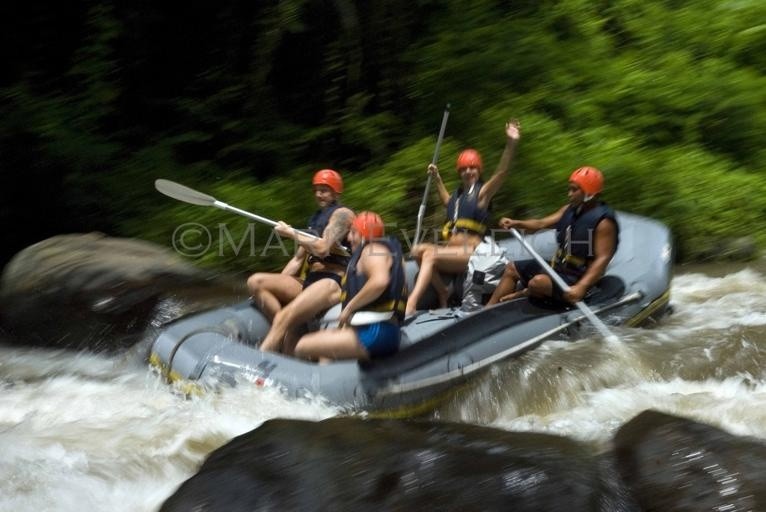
[{"x1": 147, "y1": 208, "x2": 675, "y2": 427}]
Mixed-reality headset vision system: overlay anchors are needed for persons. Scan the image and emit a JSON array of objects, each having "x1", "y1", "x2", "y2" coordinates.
[
  {"x1": 482, "y1": 165, "x2": 620, "y2": 306},
  {"x1": 249, "y1": 168, "x2": 406, "y2": 362},
  {"x1": 407, "y1": 116, "x2": 523, "y2": 322}
]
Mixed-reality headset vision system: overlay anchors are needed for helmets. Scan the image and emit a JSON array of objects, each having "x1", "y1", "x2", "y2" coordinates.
[
  {"x1": 312, "y1": 169, "x2": 344, "y2": 194},
  {"x1": 351, "y1": 211, "x2": 384, "y2": 242},
  {"x1": 568, "y1": 165, "x2": 605, "y2": 203},
  {"x1": 456, "y1": 148, "x2": 483, "y2": 172}
]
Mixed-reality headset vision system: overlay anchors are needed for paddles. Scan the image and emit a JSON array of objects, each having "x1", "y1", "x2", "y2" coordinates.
[
  {"x1": 155, "y1": 179, "x2": 350, "y2": 253},
  {"x1": 409, "y1": 103, "x2": 452, "y2": 260}
]
